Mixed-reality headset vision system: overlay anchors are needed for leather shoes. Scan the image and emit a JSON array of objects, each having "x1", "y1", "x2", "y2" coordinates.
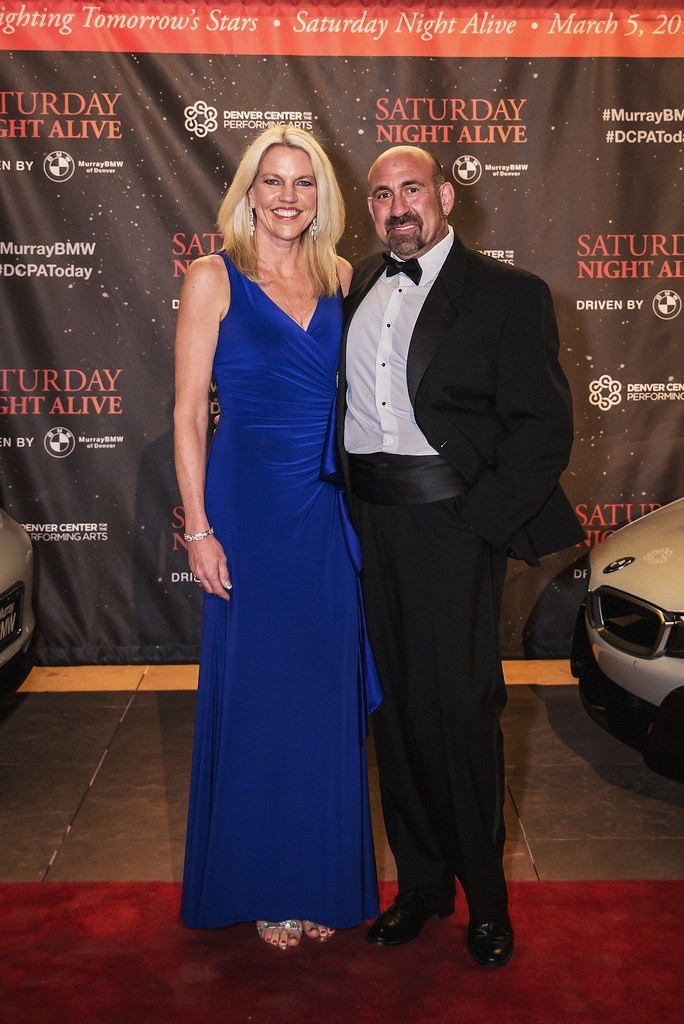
[
  {"x1": 469, "y1": 904, "x2": 513, "y2": 966},
  {"x1": 370, "y1": 888, "x2": 455, "y2": 945}
]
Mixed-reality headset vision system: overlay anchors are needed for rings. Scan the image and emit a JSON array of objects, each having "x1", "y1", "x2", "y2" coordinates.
[{"x1": 194, "y1": 577, "x2": 202, "y2": 582}]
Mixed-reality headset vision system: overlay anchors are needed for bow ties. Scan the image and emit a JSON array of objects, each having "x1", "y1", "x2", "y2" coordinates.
[{"x1": 385, "y1": 252, "x2": 423, "y2": 286}]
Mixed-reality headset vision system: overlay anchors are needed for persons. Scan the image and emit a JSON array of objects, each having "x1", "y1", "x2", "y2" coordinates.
[
  {"x1": 169, "y1": 123, "x2": 367, "y2": 952},
  {"x1": 212, "y1": 146, "x2": 578, "y2": 971}
]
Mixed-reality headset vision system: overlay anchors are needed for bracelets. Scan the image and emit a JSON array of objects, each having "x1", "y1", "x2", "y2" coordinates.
[{"x1": 184, "y1": 527, "x2": 214, "y2": 542}]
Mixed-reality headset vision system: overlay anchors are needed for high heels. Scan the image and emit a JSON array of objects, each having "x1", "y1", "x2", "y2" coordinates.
[{"x1": 255, "y1": 919, "x2": 335, "y2": 949}]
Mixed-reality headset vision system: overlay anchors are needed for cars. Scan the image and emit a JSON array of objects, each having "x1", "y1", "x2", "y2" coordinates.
[
  {"x1": 0, "y1": 508, "x2": 37, "y2": 673},
  {"x1": 569, "y1": 497, "x2": 684, "y2": 784}
]
